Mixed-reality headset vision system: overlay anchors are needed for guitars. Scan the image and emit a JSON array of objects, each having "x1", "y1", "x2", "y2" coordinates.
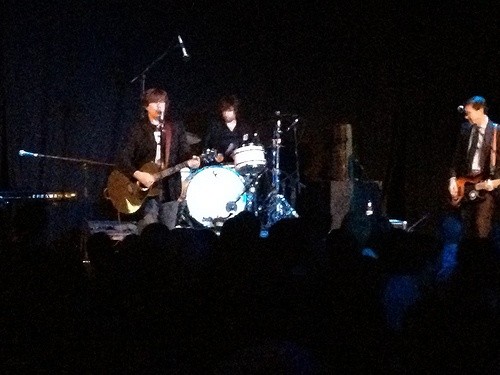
[
  {"x1": 449, "y1": 173, "x2": 500, "y2": 205},
  {"x1": 105, "y1": 148, "x2": 216, "y2": 216}
]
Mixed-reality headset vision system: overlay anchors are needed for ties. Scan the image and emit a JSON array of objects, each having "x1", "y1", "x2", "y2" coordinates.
[{"x1": 468, "y1": 127, "x2": 478, "y2": 175}]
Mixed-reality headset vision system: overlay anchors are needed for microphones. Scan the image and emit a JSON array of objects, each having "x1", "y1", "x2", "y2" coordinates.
[
  {"x1": 456, "y1": 105, "x2": 468, "y2": 115},
  {"x1": 18, "y1": 149, "x2": 39, "y2": 157},
  {"x1": 178, "y1": 34, "x2": 190, "y2": 62}
]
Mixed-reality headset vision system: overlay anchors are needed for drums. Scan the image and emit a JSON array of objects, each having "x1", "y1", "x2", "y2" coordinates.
[
  {"x1": 179, "y1": 165, "x2": 247, "y2": 229},
  {"x1": 230, "y1": 142, "x2": 267, "y2": 173}
]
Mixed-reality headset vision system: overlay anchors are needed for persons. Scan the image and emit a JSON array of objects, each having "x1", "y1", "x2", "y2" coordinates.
[
  {"x1": 0, "y1": 208, "x2": 500, "y2": 375},
  {"x1": 444, "y1": 96, "x2": 500, "y2": 238},
  {"x1": 203, "y1": 93, "x2": 260, "y2": 166},
  {"x1": 119, "y1": 89, "x2": 201, "y2": 233}
]
